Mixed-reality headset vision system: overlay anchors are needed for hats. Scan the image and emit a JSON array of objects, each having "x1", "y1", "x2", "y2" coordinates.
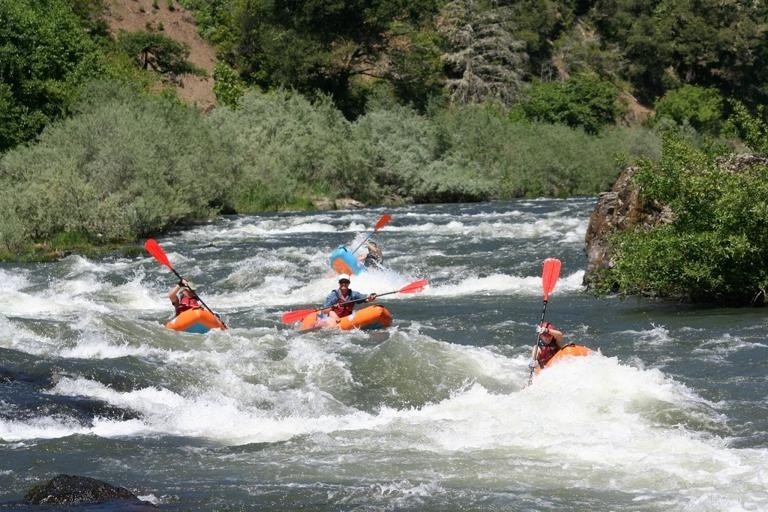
[
  {"x1": 179, "y1": 282, "x2": 197, "y2": 294},
  {"x1": 538, "y1": 322, "x2": 554, "y2": 336},
  {"x1": 365, "y1": 239, "x2": 377, "y2": 248},
  {"x1": 338, "y1": 274, "x2": 350, "y2": 284}
]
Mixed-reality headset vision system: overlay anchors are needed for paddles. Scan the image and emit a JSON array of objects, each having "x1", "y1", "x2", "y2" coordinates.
[
  {"x1": 350, "y1": 214, "x2": 392, "y2": 255},
  {"x1": 282, "y1": 280, "x2": 427, "y2": 323},
  {"x1": 144, "y1": 239, "x2": 227, "y2": 330},
  {"x1": 527, "y1": 258, "x2": 561, "y2": 387}
]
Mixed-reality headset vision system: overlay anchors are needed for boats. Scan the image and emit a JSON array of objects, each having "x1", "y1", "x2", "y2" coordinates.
[
  {"x1": 292, "y1": 303, "x2": 396, "y2": 337},
  {"x1": 160, "y1": 309, "x2": 229, "y2": 336},
  {"x1": 324, "y1": 246, "x2": 369, "y2": 276},
  {"x1": 531, "y1": 343, "x2": 603, "y2": 379}
]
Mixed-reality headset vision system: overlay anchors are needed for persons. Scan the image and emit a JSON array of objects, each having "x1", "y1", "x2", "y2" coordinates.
[
  {"x1": 356, "y1": 239, "x2": 384, "y2": 272},
  {"x1": 322, "y1": 273, "x2": 376, "y2": 319},
  {"x1": 169, "y1": 278, "x2": 204, "y2": 317},
  {"x1": 527, "y1": 323, "x2": 562, "y2": 369}
]
